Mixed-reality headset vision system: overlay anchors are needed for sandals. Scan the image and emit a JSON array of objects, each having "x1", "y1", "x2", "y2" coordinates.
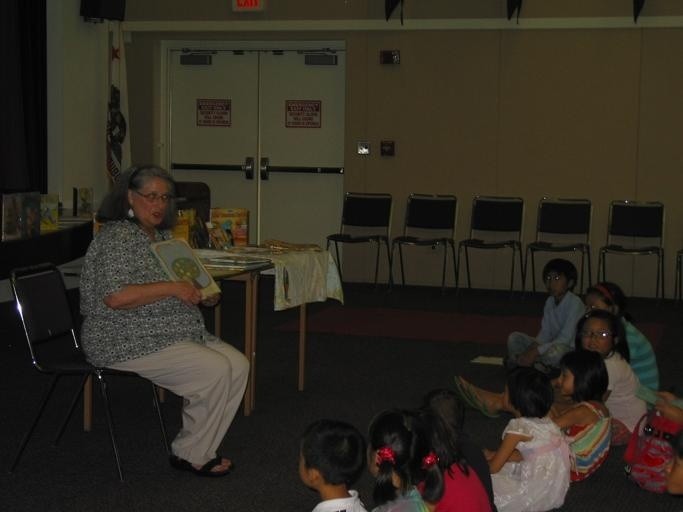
[{"x1": 169, "y1": 454, "x2": 235, "y2": 476}]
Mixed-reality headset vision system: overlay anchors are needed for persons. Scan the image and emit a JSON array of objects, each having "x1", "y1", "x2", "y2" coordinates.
[
  {"x1": 506, "y1": 259, "x2": 586, "y2": 375},
  {"x1": 653, "y1": 389, "x2": 682, "y2": 424},
  {"x1": 79, "y1": 164, "x2": 251, "y2": 477},
  {"x1": 547, "y1": 350, "x2": 612, "y2": 484},
  {"x1": 551, "y1": 309, "x2": 648, "y2": 447},
  {"x1": 422, "y1": 389, "x2": 493, "y2": 512},
  {"x1": 299, "y1": 419, "x2": 367, "y2": 512},
  {"x1": 653, "y1": 428, "x2": 683, "y2": 494},
  {"x1": 453, "y1": 281, "x2": 660, "y2": 418},
  {"x1": 366, "y1": 408, "x2": 446, "y2": 512},
  {"x1": 478, "y1": 367, "x2": 571, "y2": 512}
]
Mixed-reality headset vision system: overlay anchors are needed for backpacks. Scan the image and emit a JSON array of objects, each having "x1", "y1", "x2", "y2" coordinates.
[{"x1": 622, "y1": 411, "x2": 683, "y2": 492}]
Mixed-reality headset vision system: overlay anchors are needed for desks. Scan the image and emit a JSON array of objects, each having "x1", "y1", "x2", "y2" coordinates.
[{"x1": 58, "y1": 242, "x2": 327, "y2": 434}]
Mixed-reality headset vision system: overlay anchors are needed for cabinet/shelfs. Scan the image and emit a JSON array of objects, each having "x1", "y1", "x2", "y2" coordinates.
[{"x1": 0, "y1": 208, "x2": 93, "y2": 302}]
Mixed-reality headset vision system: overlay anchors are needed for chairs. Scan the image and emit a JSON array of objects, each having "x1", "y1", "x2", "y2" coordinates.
[
  {"x1": 457, "y1": 194, "x2": 523, "y2": 291},
  {"x1": 390, "y1": 192, "x2": 457, "y2": 287},
  {"x1": 598, "y1": 199, "x2": 667, "y2": 298},
  {"x1": 523, "y1": 197, "x2": 593, "y2": 297},
  {"x1": 673, "y1": 247, "x2": 683, "y2": 315},
  {"x1": 6, "y1": 261, "x2": 173, "y2": 481},
  {"x1": 325, "y1": 192, "x2": 393, "y2": 283}
]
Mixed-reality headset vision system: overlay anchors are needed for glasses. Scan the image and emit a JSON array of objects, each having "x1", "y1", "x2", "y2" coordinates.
[
  {"x1": 584, "y1": 303, "x2": 608, "y2": 312},
  {"x1": 133, "y1": 189, "x2": 170, "y2": 201},
  {"x1": 545, "y1": 275, "x2": 561, "y2": 282},
  {"x1": 579, "y1": 329, "x2": 612, "y2": 338}
]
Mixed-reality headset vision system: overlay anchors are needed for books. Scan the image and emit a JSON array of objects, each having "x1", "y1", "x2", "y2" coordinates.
[
  {"x1": 73, "y1": 186, "x2": 95, "y2": 217},
  {"x1": 0, "y1": 191, "x2": 59, "y2": 243},
  {"x1": 193, "y1": 240, "x2": 321, "y2": 271},
  {"x1": 147, "y1": 237, "x2": 222, "y2": 300}
]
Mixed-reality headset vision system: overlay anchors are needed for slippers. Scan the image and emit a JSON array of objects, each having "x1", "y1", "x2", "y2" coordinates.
[
  {"x1": 453, "y1": 372, "x2": 481, "y2": 410},
  {"x1": 455, "y1": 379, "x2": 503, "y2": 419}
]
